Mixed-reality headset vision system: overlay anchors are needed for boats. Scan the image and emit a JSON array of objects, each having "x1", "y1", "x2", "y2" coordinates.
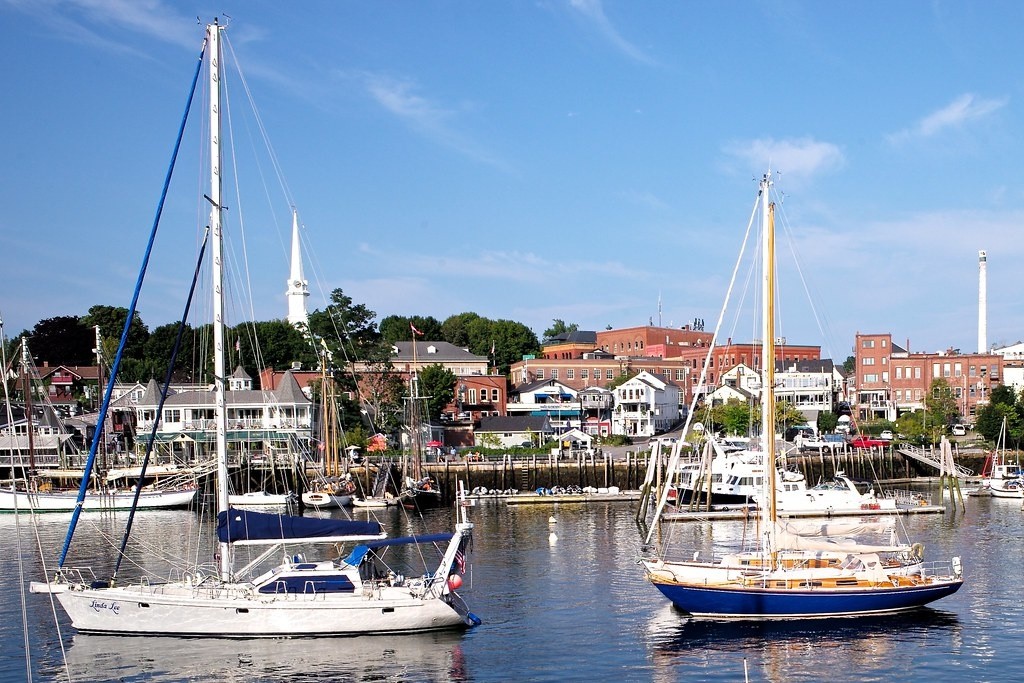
[{"x1": 652, "y1": 412, "x2": 1024, "y2": 524}]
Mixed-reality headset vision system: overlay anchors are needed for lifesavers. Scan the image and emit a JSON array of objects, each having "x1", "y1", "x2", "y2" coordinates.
[{"x1": 911, "y1": 542, "x2": 923, "y2": 558}]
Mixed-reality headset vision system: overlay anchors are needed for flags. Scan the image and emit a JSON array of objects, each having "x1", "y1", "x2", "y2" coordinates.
[{"x1": 454, "y1": 537, "x2": 465, "y2": 575}]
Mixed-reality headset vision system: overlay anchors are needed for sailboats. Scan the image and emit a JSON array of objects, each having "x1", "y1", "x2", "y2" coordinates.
[
  {"x1": 21, "y1": 13, "x2": 487, "y2": 636},
  {"x1": 644, "y1": 163, "x2": 974, "y2": 618},
  {"x1": 0, "y1": 320, "x2": 442, "y2": 511}
]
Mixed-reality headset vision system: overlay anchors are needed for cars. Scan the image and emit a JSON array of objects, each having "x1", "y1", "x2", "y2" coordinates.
[
  {"x1": 952, "y1": 423, "x2": 966, "y2": 436},
  {"x1": 879, "y1": 429, "x2": 893, "y2": 440},
  {"x1": 897, "y1": 432, "x2": 908, "y2": 440},
  {"x1": 915, "y1": 434, "x2": 939, "y2": 448}
]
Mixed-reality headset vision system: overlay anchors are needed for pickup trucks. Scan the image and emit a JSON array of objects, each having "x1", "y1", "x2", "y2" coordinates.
[
  {"x1": 799, "y1": 436, "x2": 843, "y2": 453},
  {"x1": 852, "y1": 436, "x2": 890, "y2": 452}
]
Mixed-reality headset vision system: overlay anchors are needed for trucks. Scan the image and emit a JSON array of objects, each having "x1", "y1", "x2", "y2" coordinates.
[{"x1": 834, "y1": 415, "x2": 851, "y2": 435}]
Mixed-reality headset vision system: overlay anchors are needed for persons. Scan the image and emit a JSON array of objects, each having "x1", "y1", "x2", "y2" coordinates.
[
  {"x1": 310, "y1": 477, "x2": 353, "y2": 492},
  {"x1": 417, "y1": 477, "x2": 430, "y2": 491},
  {"x1": 266, "y1": 447, "x2": 270, "y2": 454},
  {"x1": 425, "y1": 572, "x2": 434, "y2": 588},
  {"x1": 375, "y1": 570, "x2": 405, "y2": 587}
]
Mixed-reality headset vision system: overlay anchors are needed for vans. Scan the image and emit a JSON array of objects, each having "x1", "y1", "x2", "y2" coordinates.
[
  {"x1": 648, "y1": 437, "x2": 676, "y2": 448},
  {"x1": 782, "y1": 426, "x2": 814, "y2": 440}
]
